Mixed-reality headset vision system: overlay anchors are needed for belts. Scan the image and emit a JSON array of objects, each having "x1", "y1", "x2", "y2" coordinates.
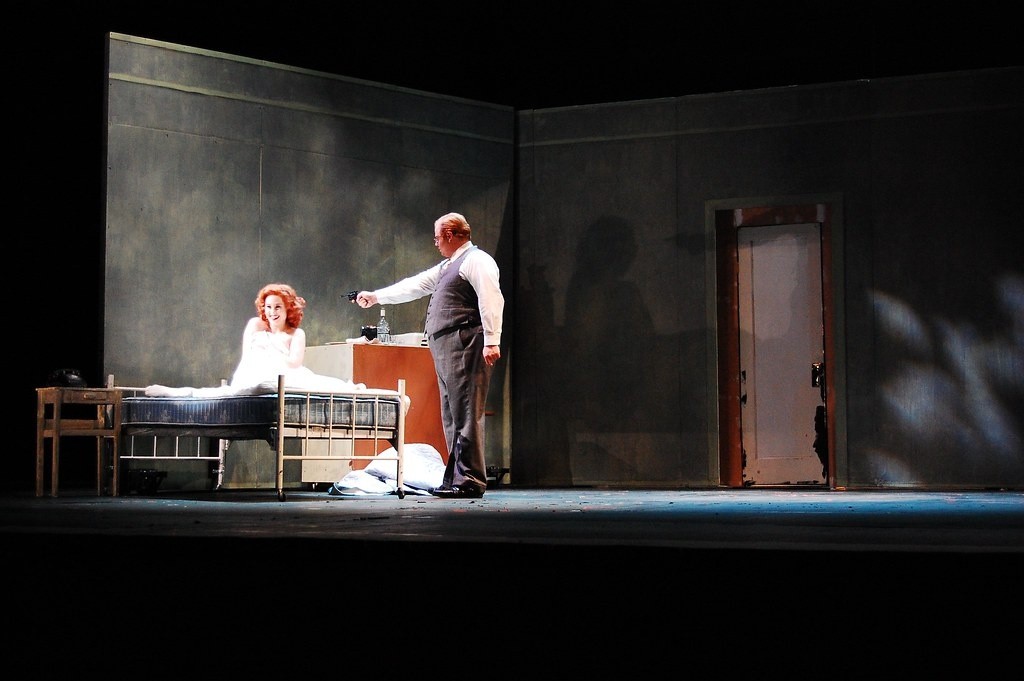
[{"x1": 428, "y1": 321, "x2": 482, "y2": 341}]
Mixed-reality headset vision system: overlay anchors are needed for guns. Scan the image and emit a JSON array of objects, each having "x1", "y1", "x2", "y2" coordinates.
[{"x1": 339, "y1": 290, "x2": 358, "y2": 302}]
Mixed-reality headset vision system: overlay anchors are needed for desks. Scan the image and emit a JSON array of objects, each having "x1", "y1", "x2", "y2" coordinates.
[{"x1": 36, "y1": 387, "x2": 123, "y2": 498}]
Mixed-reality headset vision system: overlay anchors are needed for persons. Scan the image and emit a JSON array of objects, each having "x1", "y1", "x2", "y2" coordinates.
[
  {"x1": 350, "y1": 213, "x2": 506, "y2": 498},
  {"x1": 218, "y1": 284, "x2": 306, "y2": 386}
]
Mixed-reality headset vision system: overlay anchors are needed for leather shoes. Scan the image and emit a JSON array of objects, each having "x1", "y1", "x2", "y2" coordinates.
[{"x1": 427, "y1": 485, "x2": 483, "y2": 498}]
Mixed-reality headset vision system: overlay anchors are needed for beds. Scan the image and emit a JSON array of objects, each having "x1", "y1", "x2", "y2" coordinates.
[{"x1": 108, "y1": 375, "x2": 410, "y2": 501}]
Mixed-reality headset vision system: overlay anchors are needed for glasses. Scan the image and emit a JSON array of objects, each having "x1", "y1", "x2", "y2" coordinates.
[{"x1": 433, "y1": 232, "x2": 446, "y2": 243}]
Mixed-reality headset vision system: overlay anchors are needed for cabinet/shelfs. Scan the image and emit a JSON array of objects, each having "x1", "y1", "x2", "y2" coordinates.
[{"x1": 301, "y1": 344, "x2": 448, "y2": 480}]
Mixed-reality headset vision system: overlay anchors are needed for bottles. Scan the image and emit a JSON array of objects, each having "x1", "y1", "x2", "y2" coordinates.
[{"x1": 377, "y1": 308, "x2": 389, "y2": 343}]
